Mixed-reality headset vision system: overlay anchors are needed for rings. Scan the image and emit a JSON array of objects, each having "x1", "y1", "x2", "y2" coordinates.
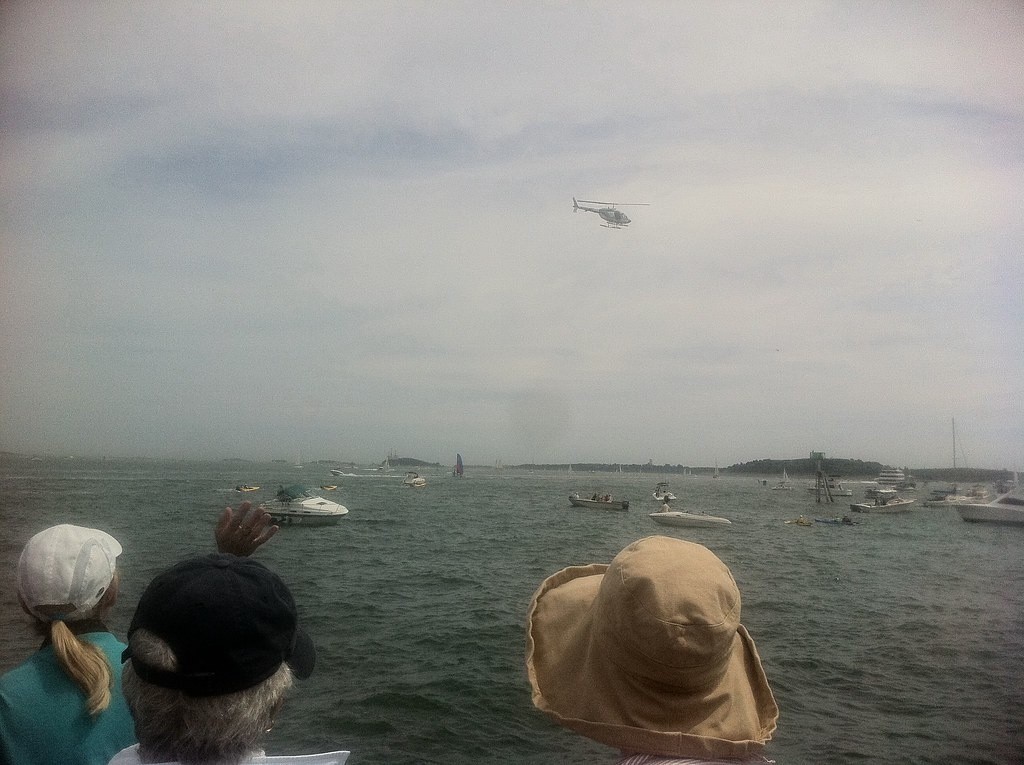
[{"x1": 239, "y1": 526, "x2": 252, "y2": 535}]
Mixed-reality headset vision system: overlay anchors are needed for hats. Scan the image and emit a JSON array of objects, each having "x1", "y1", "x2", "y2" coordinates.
[
  {"x1": 524, "y1": 536, "x2": 778, "y2": 760},
  {"x1": 121, "y1": 553, "x2": 317, "y2": 695},
  {"x1": 17, "y1": 524, "x2": 122, "y2": 622}
]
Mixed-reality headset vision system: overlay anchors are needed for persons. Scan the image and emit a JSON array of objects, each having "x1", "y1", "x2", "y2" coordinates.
[
  {"x1": 798, "y1": 495, "x2": 886, "y2": 524},
  {"x1": 525, "y1": 537, "x2": 779, "y2": 765},
  {"x1": 241, "y1": 484, "x2": 248, "y2": 489},
  {"x1": 656, "y1": 488, "x2": 671, "y2": 513},
  {"x1": 108, "y1": 554, "x2": 353, "y2": 765},
  {"x1": 0, "y1": 501, "x2": 280, "y2": 765},
  {"x1": 591, "y1": 493, "x2": 612, "y2": 502}
]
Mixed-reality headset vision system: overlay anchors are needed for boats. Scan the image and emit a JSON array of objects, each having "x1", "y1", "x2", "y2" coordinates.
[
  {"x1": 234, "y1": 486, "x2": 260, "y2": 493},
  {"x1": 328, "y1": 469, "x2": 345, "y2": 478},
  {"x1": 862, "y1": 465, "x2": 916, "y2": 501},
  {"x1": 925, "y1": 495, "x2": 975, "y2": 507},
  {"x1": 815, "y1": 517, "x2": 856, "y2": 525},
  {"x1": 807, "y1": 478, "x2": 853, "y2": 497},
  {"x1": 257, "y1": 485, "x2": 350, "y2": 526},
  {"x1": 965, "y1": 483, "x2": 992, "y2": 499},
  {"x1": 400, "y1": 472, "x2": 427, "y2": 488},
  {"x1": 652, "y1": 481, "x2": 677, "y2": 502},
  {"x1": 850, "y1": 493, "x2": 917, "y2": 513},
  {"x1": 760, "y1": 478, "x2": 768, "y2": 482},
  {"x1": 952, "y1": 481, "x2": 1024, "y2": 524},
  {"x1": 647, "y1": 510, "x2": 731, "y2": 529},
  {"x1": 318, "y1": 485, "x2": 338, "y2": 492},
  {"x1": 993, "y1": 480, "x2": 1017, "y2": 493},
  {"x1": 568, "y1": 495, "x2": 630, "y2": 508},
  {"x1": 770, "y1": 484, "x2": 792, "y2": 491}
]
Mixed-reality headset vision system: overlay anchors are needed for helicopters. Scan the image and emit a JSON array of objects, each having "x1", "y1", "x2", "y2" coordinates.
[{"x1": 571, "y1": 195, "x2": 651, "y2": 229}]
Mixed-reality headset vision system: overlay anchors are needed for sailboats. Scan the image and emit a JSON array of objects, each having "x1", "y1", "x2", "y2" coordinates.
[
  {"x1": 451, "y1": 453, "x2": 465, "y2": 480},
  {"x1": 776, "y1": 469, "x2": 790, "y2": 483},
  {"x1": 930, "y1": 416, "x2": 972, "y2": 497}
]
